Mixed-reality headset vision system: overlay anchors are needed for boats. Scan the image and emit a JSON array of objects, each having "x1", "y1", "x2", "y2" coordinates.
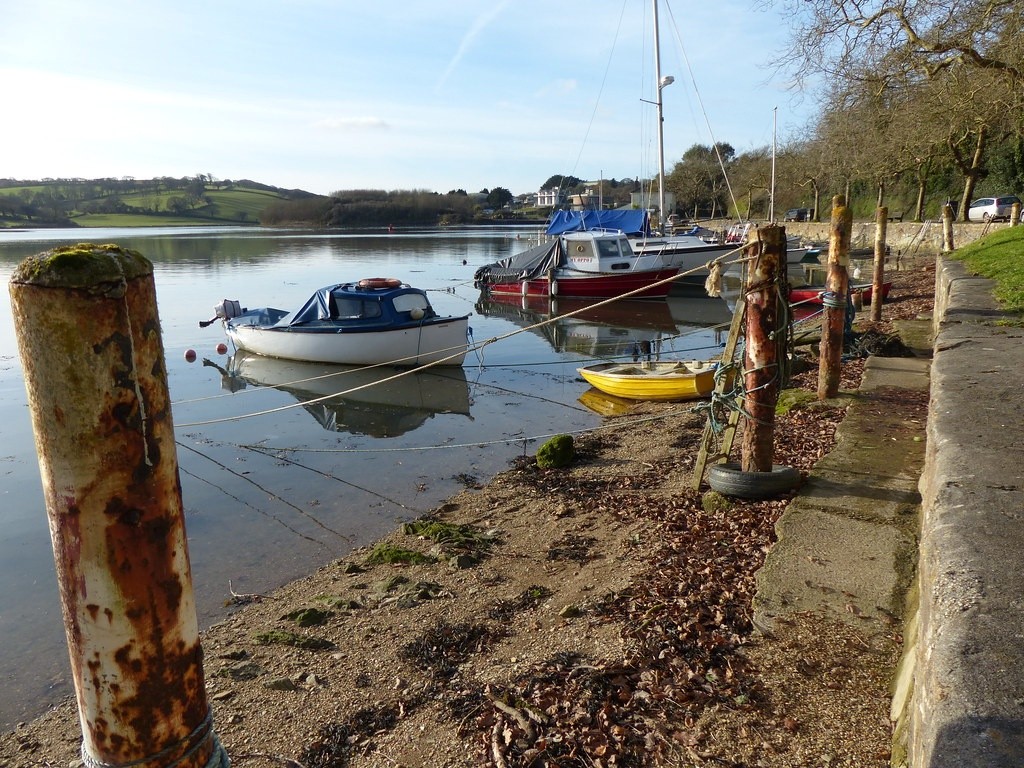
[
  {"x1": 576, "y1": 360, "x2": 744, "y2": 402},
  {"x1": 650, "y1": 106, "x2": 830, "y2": 266},
  {"x1": 787, "y1": 281, "x2": 893, "y2": 306},
  {"x1": 474, "y1": 200, "x2": 685, "y2": 300},
  {"x1": 199, "y1": 276, "x2": 475, "y2": 367},
  {"x1": 576, "y1": 387, "x2": 636, "y2": 422},
  {"x1": 474, "y1": 291, "x2": 682, "y2": 363},
  {"x1": 201, "y1": 347, "x2": 476, "y2": 439}
]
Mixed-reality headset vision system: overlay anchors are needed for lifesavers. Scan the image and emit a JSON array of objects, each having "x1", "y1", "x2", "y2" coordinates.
[{"x1": 359, "y1": 277, "x2": 402, "y2": 289}]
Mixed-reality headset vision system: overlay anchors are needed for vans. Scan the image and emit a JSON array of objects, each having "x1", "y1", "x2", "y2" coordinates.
[{"x1": 783, "y1": 208, "x2": 814, "y2": 222}]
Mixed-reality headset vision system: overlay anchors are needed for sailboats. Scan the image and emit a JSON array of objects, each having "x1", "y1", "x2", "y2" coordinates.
[{"x1": 536, "y1": 0, "x2": 752, "y2": 284}]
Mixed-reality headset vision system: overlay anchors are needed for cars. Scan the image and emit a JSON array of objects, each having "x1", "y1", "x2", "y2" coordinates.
[{"x1": 968, "y1": 195, "x2": 1023, "y2": 223}]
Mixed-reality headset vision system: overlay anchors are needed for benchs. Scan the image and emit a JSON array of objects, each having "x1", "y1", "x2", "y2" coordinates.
[
  {"x1": 887, "y1": 211, "x2": 905, "y2": 222},
  {"x1": 647, "y1": 366, "x2": 686, "y2": 375},
  {"x1": 598, "y1": 366, "x2": 636, "y2": 374}
]
